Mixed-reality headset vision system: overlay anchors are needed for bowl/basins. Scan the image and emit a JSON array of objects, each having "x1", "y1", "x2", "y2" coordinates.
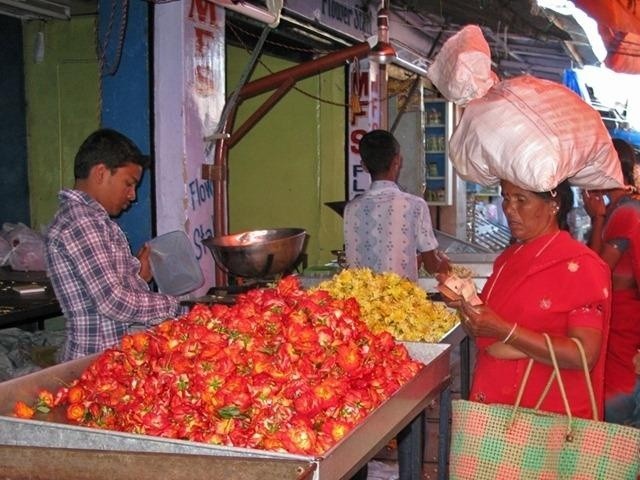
[{"x1": 199, "y1": 227, "x2": 308, "y2": 278}]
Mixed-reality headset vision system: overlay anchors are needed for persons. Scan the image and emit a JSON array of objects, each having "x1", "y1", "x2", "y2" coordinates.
[
  {"x1": 43, "y1": 127, "x2": 179, "y2": 364},
  {"x1": 432, "y1": 176, "x2": 613, "y2": 427},
  {"x1": 342, "y1": 128, "x2": 452, "y2": 286},
  {"x1": 631, "y1": 153, "x2": 640, "y2": 191},
  {"x1": 578, "y1": 137, "x2": 640, "y2": 422}
]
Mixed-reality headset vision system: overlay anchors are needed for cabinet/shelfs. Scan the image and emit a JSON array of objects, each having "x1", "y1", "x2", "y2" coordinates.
[{"x1": 423, "y1": 97, "x2": 453, "y2": 207}]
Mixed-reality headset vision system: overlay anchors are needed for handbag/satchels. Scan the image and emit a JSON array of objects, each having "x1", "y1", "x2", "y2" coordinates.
[{"x1": 448, "y1": 332, "x2": 640, "y2": 480}]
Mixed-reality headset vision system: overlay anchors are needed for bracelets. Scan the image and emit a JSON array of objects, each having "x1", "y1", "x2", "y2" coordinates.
[
  {"x1": 503, "y1": 322, "x2": 524, "y2": 346},
  {"x1": 591, "y1": 215, "x2": 605, "y2": 223}
]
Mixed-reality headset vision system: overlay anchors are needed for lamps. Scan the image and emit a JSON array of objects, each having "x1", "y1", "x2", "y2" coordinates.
[{"x1": 369, "y1": 1, "x2": 398, "y2": 65}]
